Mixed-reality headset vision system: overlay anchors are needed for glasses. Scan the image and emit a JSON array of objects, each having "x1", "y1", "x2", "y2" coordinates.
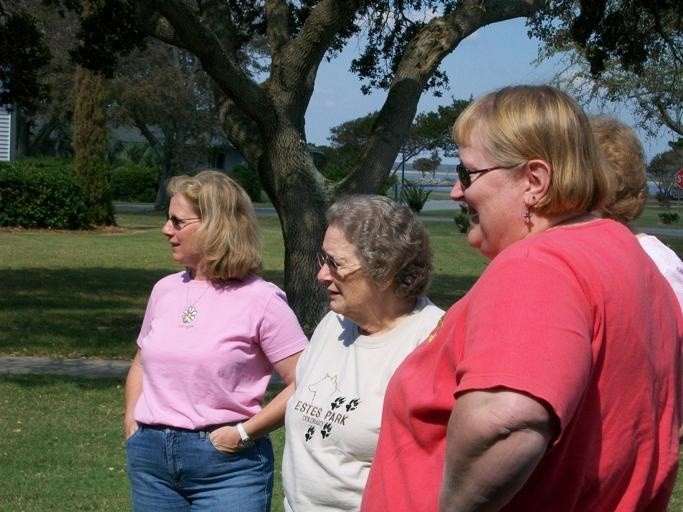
[
  {"x1": 456, "y1": 162, "x2": 525, "y2": 187},
  {"x1": 165, "y1": 211, "x2": 201, "y2": 227},
  {"x1": 316, "y1": 251, "x2": 366, "y2": 276}
]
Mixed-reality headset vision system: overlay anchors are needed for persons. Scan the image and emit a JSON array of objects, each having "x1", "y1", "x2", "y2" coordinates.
[
  {"x1": 363, "y1": 85, "x2": 681, "y2": 512},
  {"x1": 122, "y1": 170, "x2": 311, "y2": 511},
  {"x1": 589, "y1": 114, "x2": 680, "y2": 310},
  {"x1": 282, "y1": 194, "x2": 450, "y2": 512}
]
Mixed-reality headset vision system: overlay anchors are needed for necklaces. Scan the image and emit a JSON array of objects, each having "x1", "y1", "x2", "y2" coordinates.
[{"x1": 182, "y1": 271, "x2": 213, "y2": 323}]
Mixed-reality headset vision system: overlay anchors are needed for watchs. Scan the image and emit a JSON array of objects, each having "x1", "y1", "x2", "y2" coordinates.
[{"x1": 236, "y1": 421, "x2": 253, "y2": 448}]
